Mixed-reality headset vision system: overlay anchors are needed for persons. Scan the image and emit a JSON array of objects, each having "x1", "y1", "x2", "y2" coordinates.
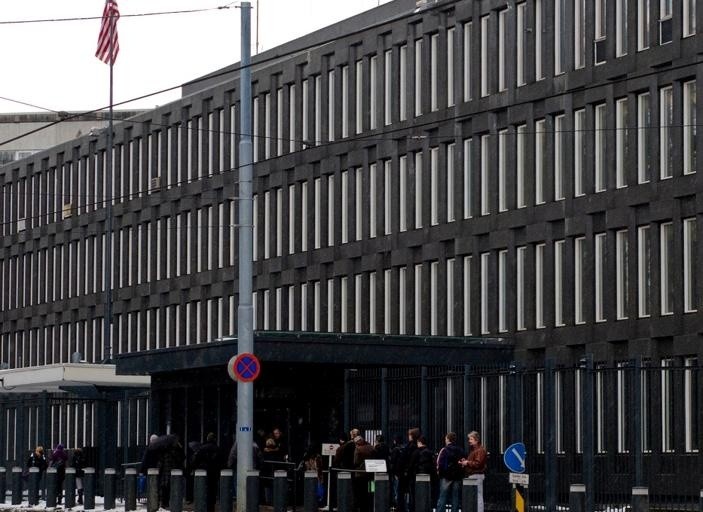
[
  {"x1": 24, "y1": 445, "x2": 49, "y2": 507},
  {"x1": 137, "y1": 424, "x2": 488, "y2": 511},
  {"x1": 47, "y1": 442, "x2": 69, "y2": 505},
  {"x1": 69, "y1": 446, "x2": 84, "y2": 505}
]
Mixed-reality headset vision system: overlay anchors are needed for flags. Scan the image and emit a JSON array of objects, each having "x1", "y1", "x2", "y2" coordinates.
[{"x1": 93, "y1": 0, "x2": 121, "y2": 68}]
